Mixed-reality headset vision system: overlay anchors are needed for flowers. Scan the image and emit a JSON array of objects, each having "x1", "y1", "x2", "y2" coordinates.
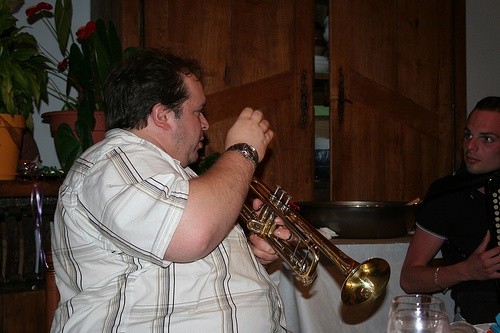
[{"x1": 26, "y1": 0, "x2": 141, "y2": 173}]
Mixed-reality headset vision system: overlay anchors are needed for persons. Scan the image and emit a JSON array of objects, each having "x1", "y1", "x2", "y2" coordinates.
[
  {"x1": 51, "y1": 46, "x2": 301, "y2": 333},
  {"x1": 399, "y1": 95, "x2": 500, "y2": 325}
]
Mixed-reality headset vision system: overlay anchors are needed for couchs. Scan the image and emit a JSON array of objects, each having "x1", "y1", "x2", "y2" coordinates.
[{"x1": 264, "y1": 201, "x2": 454, "y2": 333}]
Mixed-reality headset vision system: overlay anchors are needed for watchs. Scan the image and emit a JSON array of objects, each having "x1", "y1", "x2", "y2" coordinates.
[{"x1": 225, "y1": 143, "x2": 259, "y2": 167}]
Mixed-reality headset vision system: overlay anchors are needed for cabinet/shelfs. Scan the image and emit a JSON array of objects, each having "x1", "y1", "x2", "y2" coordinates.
[
  {"x1": 90, "y1": 0, "x2": 468, "y2": 207},
  {"x1": 0, "y1": 179, "x2": 65, "y2": 333}
]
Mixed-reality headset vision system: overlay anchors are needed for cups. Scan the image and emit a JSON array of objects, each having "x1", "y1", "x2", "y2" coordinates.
[
  {"x1": 386, "y1": 308, "x2": 451, "y2": 333},
  {"x1": 388, "y1": 294, "x2": 447, "y2": 318}
]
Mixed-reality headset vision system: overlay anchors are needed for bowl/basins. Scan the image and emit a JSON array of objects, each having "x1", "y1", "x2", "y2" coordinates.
[
  {"x1": 314, "y1": 56, "x2": 329, "y2": 73},
  {"x1": 293, "y1": 200, "x2": 422, "y2": 240}
]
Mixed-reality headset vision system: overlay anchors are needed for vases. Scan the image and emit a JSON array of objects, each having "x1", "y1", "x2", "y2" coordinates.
[{"x1": 41, "y1": 110, "x2": 106, "y2": 144}]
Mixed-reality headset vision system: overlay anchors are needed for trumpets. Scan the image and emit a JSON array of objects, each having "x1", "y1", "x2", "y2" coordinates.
[{"x1": 238, "y1": 179, "x2": 391, "y2": 308}]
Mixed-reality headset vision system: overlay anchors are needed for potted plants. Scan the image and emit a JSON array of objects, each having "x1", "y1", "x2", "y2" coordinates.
[{"x1": 0, "y1": 0, "x2": 56, "y2": 181}]
